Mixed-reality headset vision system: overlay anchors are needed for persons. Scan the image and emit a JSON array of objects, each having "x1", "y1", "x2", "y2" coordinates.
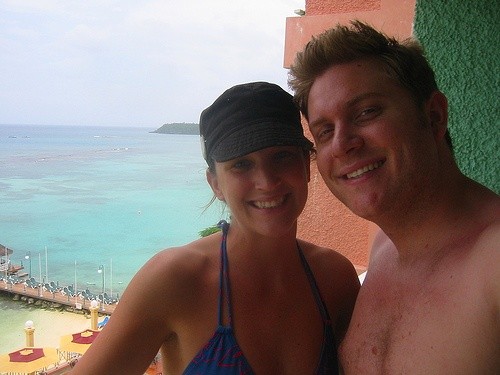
[
  {"x1": 287, "y1": 19, "x2": 500, "y2": 375},
  {"x1": 63, "y1": 81, "x2": 361, "y2": 375}
]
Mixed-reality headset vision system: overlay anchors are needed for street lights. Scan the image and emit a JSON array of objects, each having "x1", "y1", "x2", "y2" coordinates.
[
  {"x1": 24, "y1": 250, "x2": 31, "y2": 278},
  {"x1": 98, "y1": 264, "x2": 104, "y2": 293}
]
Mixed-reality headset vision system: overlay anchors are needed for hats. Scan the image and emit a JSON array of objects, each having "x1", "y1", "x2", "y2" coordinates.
[{"x1": 198, "y1": 82, "x2": 315, "y2": 165}]
